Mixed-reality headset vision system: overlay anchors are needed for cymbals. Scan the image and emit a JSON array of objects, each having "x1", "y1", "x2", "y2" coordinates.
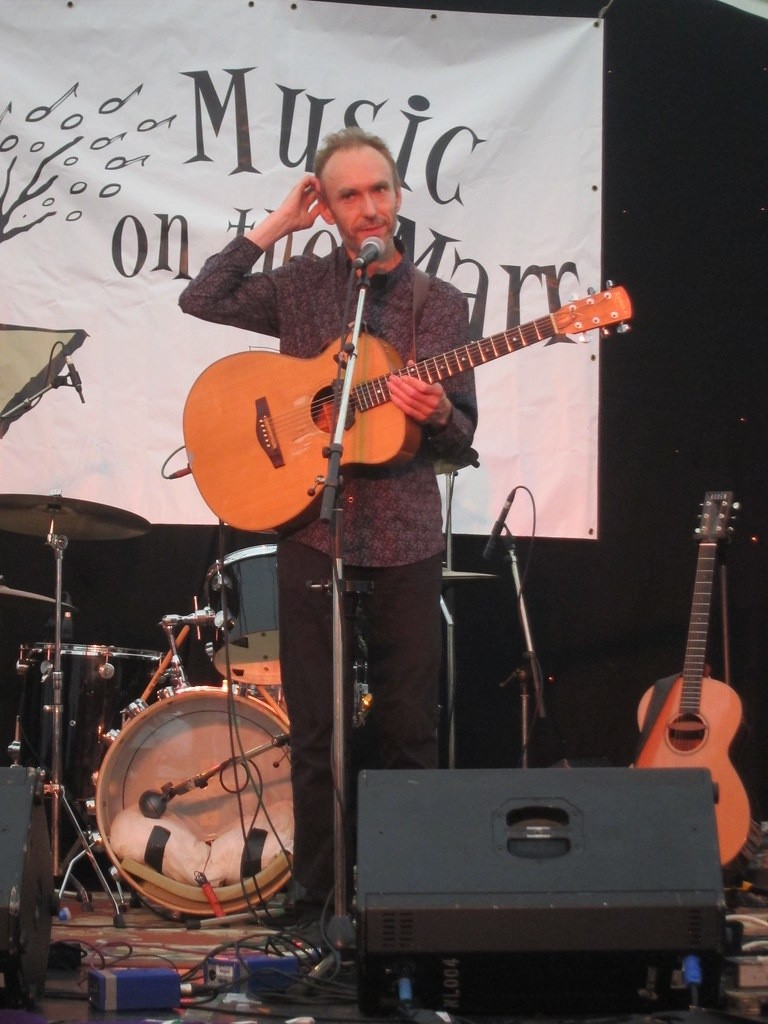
[
  {"x1": 441, "y1": 567, "x2": 499, "y2": 580},
  {"x1": 1, "y1": 584, "x2": 82, "y2": 619},
  {"x1": 2, "y1": 492, "x2": 152, "y2": 542}
]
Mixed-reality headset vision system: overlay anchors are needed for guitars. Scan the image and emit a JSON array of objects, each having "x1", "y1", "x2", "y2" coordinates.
[
  {"x1": 180, "y1": 277, "x2": 638, "y2": 544},
  {"x1": 633, "y1": 487, "x2": 753, "y2": 871}
]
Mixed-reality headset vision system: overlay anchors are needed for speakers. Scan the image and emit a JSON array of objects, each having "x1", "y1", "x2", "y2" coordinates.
[
  {"x1": 0, "y1": 767, "x2": 54, "y2": 1009},
  {"x1": 354, "y1": 765, "x2": 731, "y2": 1022}
]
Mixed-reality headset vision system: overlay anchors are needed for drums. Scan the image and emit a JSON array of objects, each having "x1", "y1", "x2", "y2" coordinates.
[
  {"x1": 95, "y1": 684, "x2": 297, "y2": 917},
  {"x1": 17, "y1": 642, "x2": 175, "y2": 805},
  {"x1": 351, "y1": 634, "x2": 369, "y2": 728},
  {"x1": 203, "y1": 543, "x2": 336, "y2": 685}
]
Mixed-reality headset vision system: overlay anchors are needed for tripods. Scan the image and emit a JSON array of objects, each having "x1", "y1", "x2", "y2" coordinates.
[{"x1": 44, "y1": 512, "x2": 127, "y2": 929}]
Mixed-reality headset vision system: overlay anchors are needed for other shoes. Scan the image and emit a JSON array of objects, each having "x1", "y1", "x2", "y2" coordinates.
[{"x1": 301, "y1": 916, "x2": 326, "y2": 947}]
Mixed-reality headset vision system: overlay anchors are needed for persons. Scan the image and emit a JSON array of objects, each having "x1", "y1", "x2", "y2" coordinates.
[{"x1": 179, "y1": 130, "x2": 478, "y2": 929}]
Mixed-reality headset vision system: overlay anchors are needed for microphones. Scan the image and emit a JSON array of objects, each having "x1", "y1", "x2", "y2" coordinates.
[
  {"x1": 482, "y1": 489, "x2": 516, "y2": 559},
  {"x1": 61, "y1": 344, "x2": 86, "y2": 404},
  {"x1": 353, "y1": 239, "x2": 384, "y2": 269}
]
